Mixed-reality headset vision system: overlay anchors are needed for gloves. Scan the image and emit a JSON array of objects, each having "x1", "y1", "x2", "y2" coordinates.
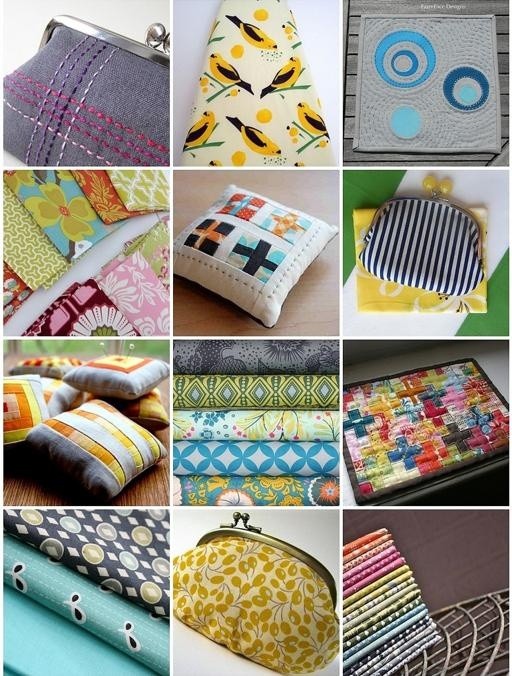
[
  {"x1": 4, "y1": 15, "x2": 170, "y2": 166},
  {"x1": 360, "y1": 176, "x2": 484, "y2": 297},
  {"x1": 171, "y1": 511, "x2": 339, "y2": 674}
]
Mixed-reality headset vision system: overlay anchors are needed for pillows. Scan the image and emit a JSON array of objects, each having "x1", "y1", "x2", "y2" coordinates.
[
  {"x1": 37, "y1": 373, "x2": 80, "y2": 418},
  {"x1": 8, "y1": 356, "x2": 83, "y2": 379},
  {"x1": 63, "y1": 353, "x2": 170, "y2": 401},
  {"x1": 28, "y1": 397, "x2": 168, "y2": 498},
  {"x1": 82, "y1": 387, "x2": 170, "y2": 429},
  {"x1": 3, "y1": 372, "x2": 49, "y2": 446},
  {"x1": 173, "y1": 182, "x2": 339, "y2": 329}
]
